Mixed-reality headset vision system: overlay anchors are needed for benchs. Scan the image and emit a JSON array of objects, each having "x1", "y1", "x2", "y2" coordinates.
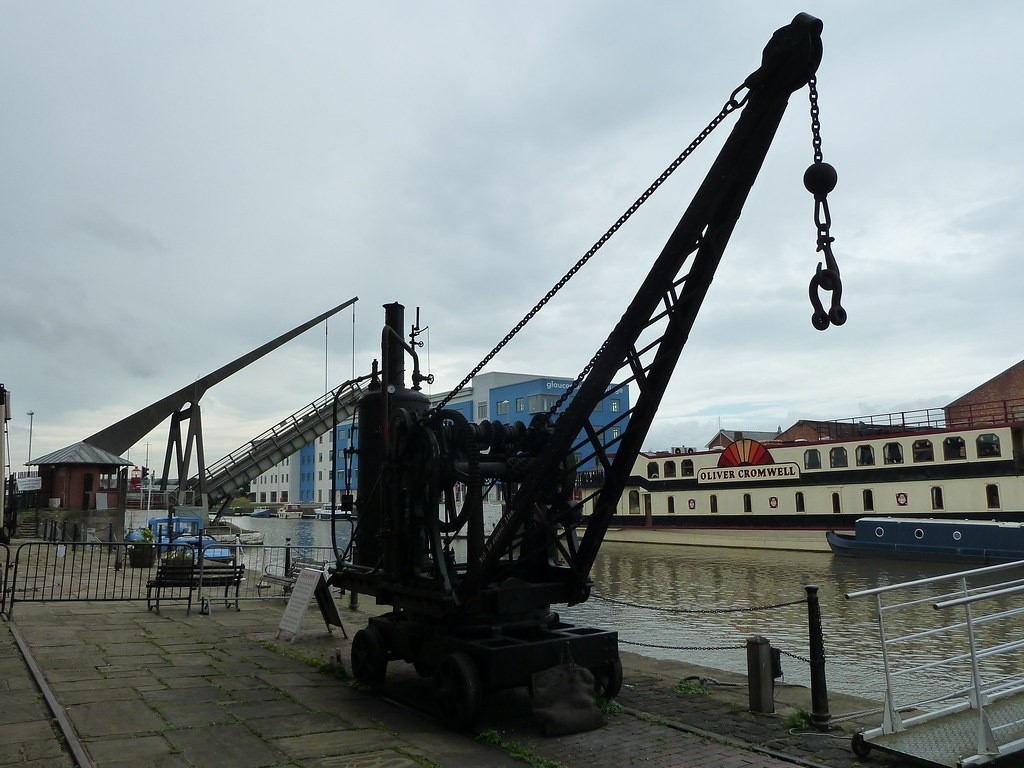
[
  {"x1": 258, "y1": 557, "x2": 328, "y2": 606},
  {"x1": 145, "y1": 564, "x2": 247, "y2": 614}
]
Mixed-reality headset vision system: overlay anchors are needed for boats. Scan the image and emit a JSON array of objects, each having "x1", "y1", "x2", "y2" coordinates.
[
  {"x1": 251, "y1": 506, "x2": 271, "y2": 518},
  {"x1": 146, "y1": 515, "x2": 237, "y2": 563},
  {"x1": 277, "y1": 503, "x2": 304, "y2": 521},
  {"x1": 313, "y1": 502, "x2": 352, "y2": 521}
]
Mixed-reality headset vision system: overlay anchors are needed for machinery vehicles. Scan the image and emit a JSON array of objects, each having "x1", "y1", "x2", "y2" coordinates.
[{"x1": 322, "y1": 16, "x2": 851, "y2": 719}]
